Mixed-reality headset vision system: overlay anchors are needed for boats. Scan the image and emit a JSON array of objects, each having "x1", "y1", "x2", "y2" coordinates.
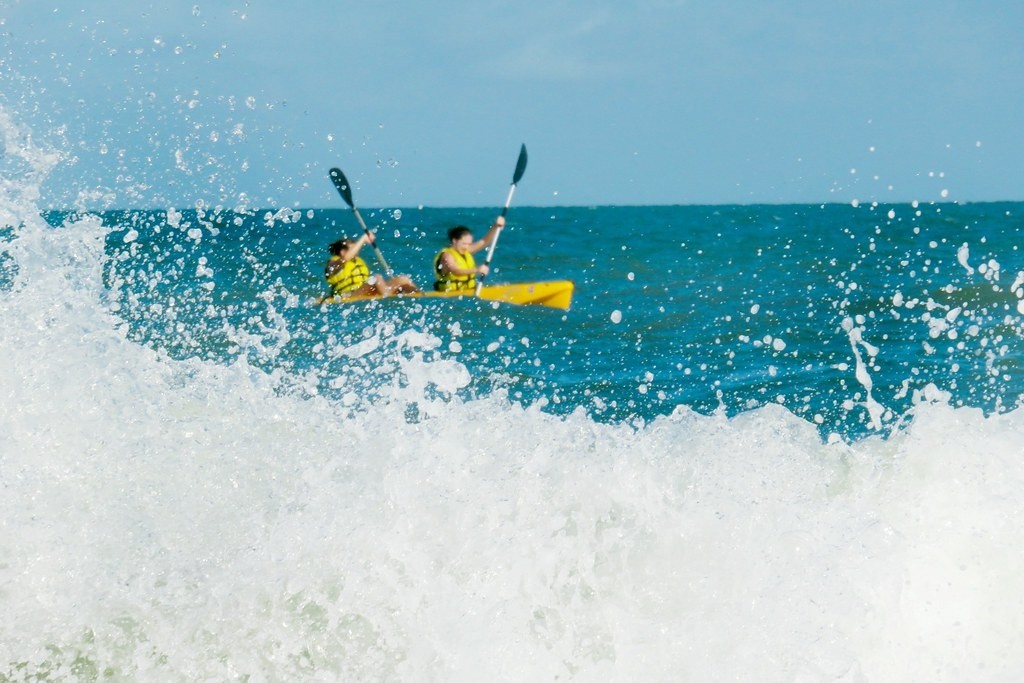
[{"x1": 306, "y1": 280, "x2": 575, "y2": 312}]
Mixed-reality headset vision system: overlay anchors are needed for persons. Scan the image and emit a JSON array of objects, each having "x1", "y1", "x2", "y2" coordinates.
[
  {"x1": 433, "y1": 217, "x2": 505, "y2": 292},
  {"x1": 323, "y1": 231, "x2": 417, "y2": 295}
]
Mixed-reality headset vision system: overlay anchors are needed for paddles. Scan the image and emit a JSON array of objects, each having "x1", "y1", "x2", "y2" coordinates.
[
  {"x1": 329, "y1": 167, "x2": 394, "y2": 280},
  {"x1": 474, "y1": 143, "x2": 528, "y2": 297}
]
